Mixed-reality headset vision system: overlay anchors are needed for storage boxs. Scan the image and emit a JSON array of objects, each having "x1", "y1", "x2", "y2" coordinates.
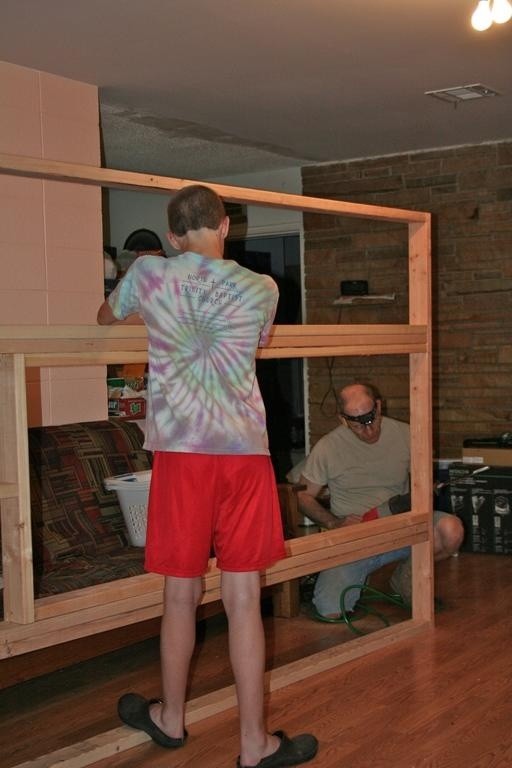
[{"x1": 447, "y1": 463, "x2": 512, "y2": 556}]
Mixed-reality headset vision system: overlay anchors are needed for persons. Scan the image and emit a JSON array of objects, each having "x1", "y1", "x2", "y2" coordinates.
[
  {"x1": 293, "y1": 383, "x2": 464, "y2": 621},
  {"x1": 98, "y1": 185, "x2": 319, "y2": 768}
]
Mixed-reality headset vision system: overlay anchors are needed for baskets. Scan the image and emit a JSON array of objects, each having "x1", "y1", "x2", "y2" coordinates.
[{"x1": 103, "y1": 470, "x2": 153, "y2": 548}]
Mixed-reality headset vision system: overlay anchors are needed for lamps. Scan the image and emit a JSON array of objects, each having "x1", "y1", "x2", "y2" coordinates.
[{"x1": 469, "y1": 1, "x2": 512, "y2": 32}]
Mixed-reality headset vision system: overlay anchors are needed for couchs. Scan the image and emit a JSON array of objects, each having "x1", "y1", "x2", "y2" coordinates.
[{"x1": 29, "y1": 420, "x2": 301, "y2": 619}]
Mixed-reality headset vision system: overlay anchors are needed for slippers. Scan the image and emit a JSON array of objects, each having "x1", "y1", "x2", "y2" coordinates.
[
  {"x1": 238, "y1": 732, "x2": 317, "y2": 767},
  {"x1": 117, "y1": 692, "x2": 186, "y2": 746}
]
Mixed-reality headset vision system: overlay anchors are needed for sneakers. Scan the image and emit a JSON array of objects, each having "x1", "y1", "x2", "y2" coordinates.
[{"x1": 390, "y1": 563, "x2": 412, "y2": 607}]
